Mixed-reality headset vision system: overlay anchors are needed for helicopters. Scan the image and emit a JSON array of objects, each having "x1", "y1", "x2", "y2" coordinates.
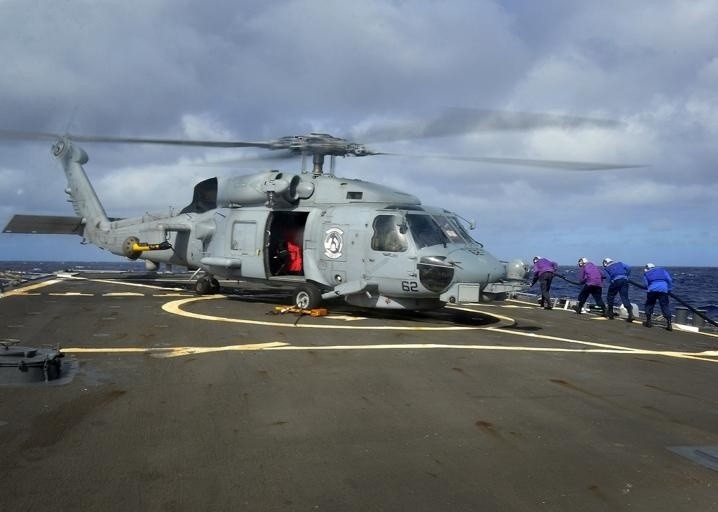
[{"x1": 0, "y1": 108, "x2": 651, "y2": 311}]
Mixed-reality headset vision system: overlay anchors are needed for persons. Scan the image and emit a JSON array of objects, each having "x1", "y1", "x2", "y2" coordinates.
[
  {"x1": 570, "y1": 256, "x2": 607, "y2": 318},
  {"x1": 528, "y1": 255, "x2": 559, "y2": 310},
  {"x1": 600, "y1": 256, "x2": 636, "y2": 323},
  {"x1": 641, "y1": 263, "x2": 673, "y2": 332}
]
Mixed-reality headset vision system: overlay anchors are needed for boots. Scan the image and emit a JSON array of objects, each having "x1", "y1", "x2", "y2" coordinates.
[
  {"x1": 572, "y1": 302, "x2": 584, "y2": 314},
  {"x1": 626, "y1": 308, "x2": 635, "y2": 322},
  {"x1": 538, "y1": 296, "x2": 545, "y2": 307},
  {"x1": 608, "y1": 307, "x2": 615, "y2": 320},
  {"x1": 600, "y1": 306, "x2": 608, "y2": 317},
  {"x1": 544, "y1": 299, "x2": 553, "y2": 309},
  {"x1": 665, "y1": 318, "x2": 673, "y2": 331},
  {"x1": 642, "y1": 316, "x2": 651, "y2": 328}
]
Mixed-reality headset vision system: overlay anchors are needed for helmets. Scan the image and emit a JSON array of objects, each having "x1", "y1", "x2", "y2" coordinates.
[
  {"x1": 533, "y1": 255, "x2": 541, "y2": 263},
  {"x1": 603, "y1": 258, "x2": 614, "y2": 267},
  {"x1": 645, "y1": 262, "x2": 656, "y2": 270},
  {"x1": 578, "y1": 258, "x2": 589, "y2": 268}
]
